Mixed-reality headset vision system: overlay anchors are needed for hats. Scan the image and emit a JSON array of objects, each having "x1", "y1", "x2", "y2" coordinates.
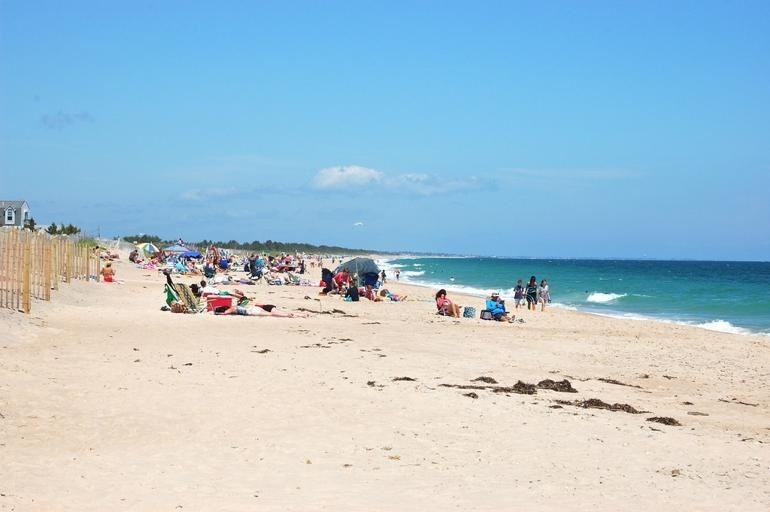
[{"x1": 491, "y1": 292, "x2": 500, "y2": 298}]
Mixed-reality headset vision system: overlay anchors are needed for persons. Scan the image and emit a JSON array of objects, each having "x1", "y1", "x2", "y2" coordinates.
[
  {"x1": 129, "y1": 238, "x2": 408, "y2": 318},
  {"x1": 100, "y1": 263, "x2": 116, "y2": 282},
  {"x1": 436, "y1": 289, "x2": 461, "y2": 318},
  {"x1": 486, "y1": 292, "x2": 515, "y2": 322},
  {"x1": 514, "y1": 276, "x2": 552, "y2": 312}
]
